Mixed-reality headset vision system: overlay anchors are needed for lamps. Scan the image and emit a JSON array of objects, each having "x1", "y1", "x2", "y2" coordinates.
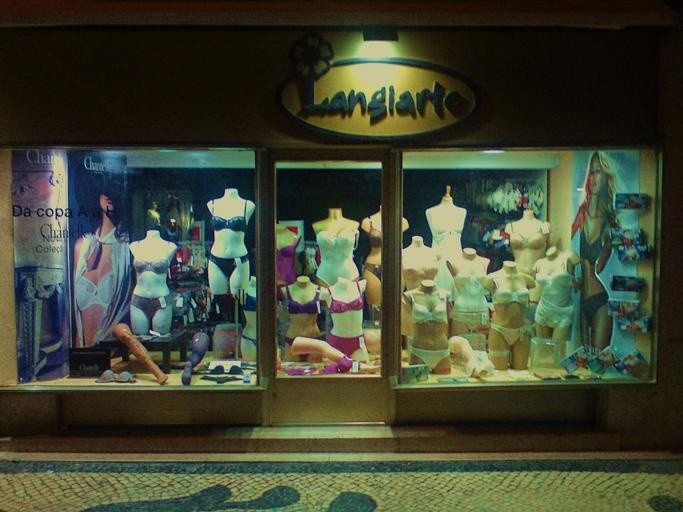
[{"x1": 357, "y1": 26, "x2": 401, "y2": 44}]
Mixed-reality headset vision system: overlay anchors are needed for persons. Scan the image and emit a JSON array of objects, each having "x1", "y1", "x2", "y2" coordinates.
[
  {"x1": 481, "y1": 259, "x2": 536, "y2": 369},
  {"x1": 309, "y1": 206, "x2": 359, "y2": 289},
  {"x1": 496, "y1": 207, "x2": 551, "y2": 279},
  {"x1": 164, "y1": 192, "x2": 182, "y2": 234},
  {"x1": 422, "y1": 196, "x2": 466, "y2": 292},
  {"x1": 145, "y1": 200, "x2": 162, "y2": 232},
  {"x1": 399, "y1": 233, "x2": 440, "y2": 336},
  {"x1": 155, "y1": 219, "x2": 199, "y2": 288},
  {"x1": 204, "y1": 186, "x2": 256, "y2": 304},
  {"x1": 356, "y1": 204, "x2": 411, "y2": 306},
  {"x1": 238, "y1": 275, "x2": 257, "y2": 363},
  {"x1": 322, "y1": 277, "x2": 369, "y2": 363},
  {"x1": 528, "y1": 243, "x2": 580, "y2": 368},
  {"x1": 68, "y1": 149, "x2": 134, "y2": 349},
  {"x1": 274, "y1": 273, "x2": 328, "y2": 362},
  {"x1": 274, "y1": 223, "x2": 302, "y2": 303},
  {"x1": 444, "y1": 245, "x2": 491, "y2": 336},
  {"x1": 402, "y1": 279, "x2": 454, "y2": 376},
  {"x1": 570, "y1": 150, "x2": 620, "y2": 356},
  {"x1": 127, "y1": 228, "x2": 178, "y2": 336}
]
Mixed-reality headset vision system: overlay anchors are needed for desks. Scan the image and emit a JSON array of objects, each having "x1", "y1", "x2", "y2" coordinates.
[{"x1": 96, "y1": 327, "x2": 188, "y2": 376}]
